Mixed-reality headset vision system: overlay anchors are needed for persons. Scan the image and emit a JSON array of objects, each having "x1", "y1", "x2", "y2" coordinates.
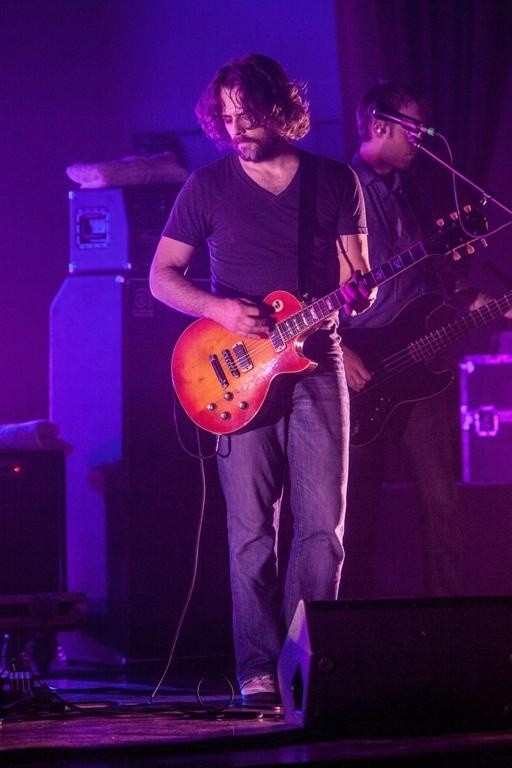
[
  {"x1": 149, "y1": 53, "x2": 380, "y2": 702},
  {"x1": 333, "y1": 86, "x2": 512, "y2": 598}
]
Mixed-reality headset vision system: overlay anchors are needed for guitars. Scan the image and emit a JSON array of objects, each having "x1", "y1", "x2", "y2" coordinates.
[
  {"x1": 171, "y1": 205, "x2": 489, "y2": 435},
  {"x1": 337, "y1": 286, "x2": 512, "y2": 447}
]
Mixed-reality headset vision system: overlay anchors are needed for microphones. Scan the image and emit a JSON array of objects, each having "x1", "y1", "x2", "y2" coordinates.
[{"x1": 370, "y1": 103, "x2": 438, "y2": 138}]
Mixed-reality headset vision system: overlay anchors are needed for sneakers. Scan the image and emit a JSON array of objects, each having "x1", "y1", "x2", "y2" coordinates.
[{"x1": 240, "y1": 672, "x2": 280, "y2": 704}]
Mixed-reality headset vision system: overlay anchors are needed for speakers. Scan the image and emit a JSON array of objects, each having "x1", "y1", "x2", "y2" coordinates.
[
  {"x1": 274, "y1": 595, "x2": 512, "y2": 730},
  {"x1": 46, "y1": 273, "x2": 234, "y2": 665},
  {"x1": 67, "y1": 185, "x2": 212, "y2": 275}
]
